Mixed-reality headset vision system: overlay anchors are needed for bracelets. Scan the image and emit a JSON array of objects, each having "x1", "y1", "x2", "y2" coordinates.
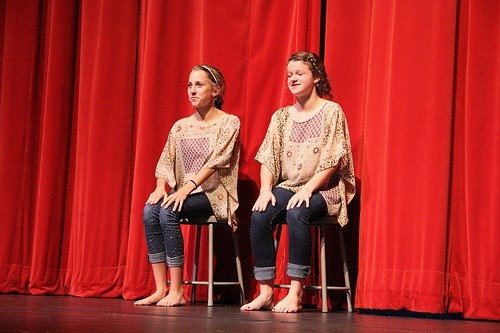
[{"x1": 190, "y1": 180, "x2": 197, "y2": 189}]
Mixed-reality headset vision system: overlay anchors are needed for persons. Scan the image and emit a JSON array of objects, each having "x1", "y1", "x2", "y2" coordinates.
[
  {"x1": 241, "y1": 51, "x2": 356, "y2": 313},
  {"x1": 134, "y1": 63, "x2": 241, "y2": 307}
]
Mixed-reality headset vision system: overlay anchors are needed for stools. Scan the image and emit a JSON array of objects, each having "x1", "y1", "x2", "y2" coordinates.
[
  {"x1": 273, "y1": 215, "x2": 353, "y2": 313},
  {"x1": 165, "y1": 212, "x2": 247, "y2": 307}
]
